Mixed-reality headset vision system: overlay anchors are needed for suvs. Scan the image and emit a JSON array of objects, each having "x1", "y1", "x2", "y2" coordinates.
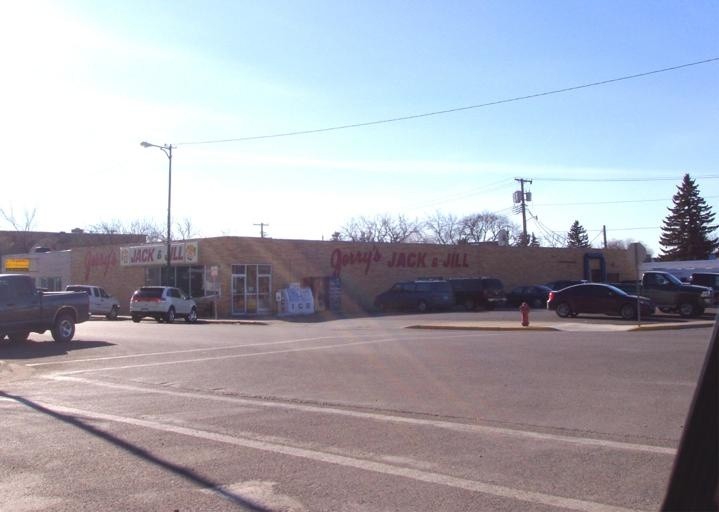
[
  {"x1": 130, "y1": 285, "x2": 199, "y2": 324},
  {"x1": 64, "y1": 284, "x2": 121, "y2": 319}
]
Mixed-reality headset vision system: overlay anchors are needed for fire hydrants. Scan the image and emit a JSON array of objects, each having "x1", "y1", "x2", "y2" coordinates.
[{"x1": 520, "y1": 302, "x2": 530, "y2": 327}]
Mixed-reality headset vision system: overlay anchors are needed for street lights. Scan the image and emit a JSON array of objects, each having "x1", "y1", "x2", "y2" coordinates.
[{"x1": 140, "y1": 141, "x2": 176, "y2": 286}]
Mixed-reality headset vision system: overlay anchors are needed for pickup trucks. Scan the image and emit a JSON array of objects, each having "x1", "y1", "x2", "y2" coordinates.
[{"x1": 1, "y1": 273, "x2": 91, "y2": 345}]
